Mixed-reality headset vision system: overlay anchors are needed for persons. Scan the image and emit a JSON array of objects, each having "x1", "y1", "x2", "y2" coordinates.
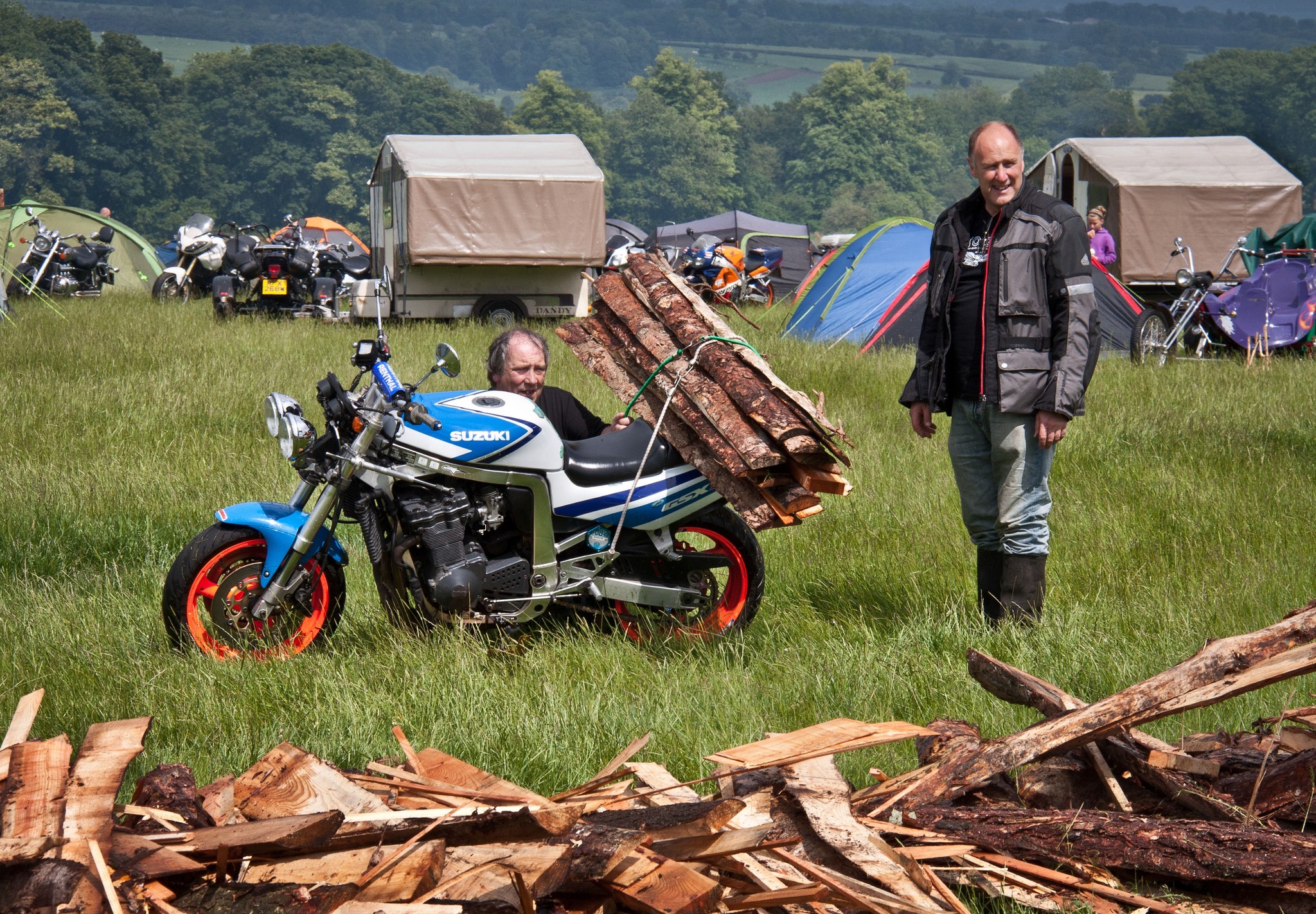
[
  {"x1": 0, "y1": 274, "x2": 16, "y2": 316},
  {"x1": 1085, "y1": 206, "x2": 1116, "y2": 268},
  {"x1": 484, "y1": 329, "x2": 634, "y2": 638},
  {"x1": 898, "y1": 121, "x2": 1101, "y2": 631},
  {"x1": 100, "y1": 207, "x2": 110, "y2": 217},
  {"x1": 189, "y1": 211, "x2": 207, "y2": 300}
]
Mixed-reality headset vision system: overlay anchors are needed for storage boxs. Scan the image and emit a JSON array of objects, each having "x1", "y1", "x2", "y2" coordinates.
[
  {"x1": 349, "y1": 278, "x2": 390, "y2": 318},
  {"x1": 235, "y1": 251, "x2": 262, "y2": 279},
  {"x1": 287, "y1": 247, "x2": 315, "y2": 276}
]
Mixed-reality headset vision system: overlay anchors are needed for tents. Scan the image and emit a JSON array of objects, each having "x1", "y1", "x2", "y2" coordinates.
[
  {"x1": 154, "y1": 231, "x2": 221, "y2": 270},
  {"x1": 266, "y1": 216, "x2": 370, "y2": 260},
  {"x1": 777, "y1": 217, "x2": 935, "y2": 358},
  {"x1": 588, "y1": 218, "x2": 649, "y2": 300},
  {"x1": 859, "y1": 255, "x2": 1142, "y2": 357},
  {"x1": 647, "y1": 210, "x2": 825, "y2": 305},
  {"x1": 0, "y1": 196, "x2": 167, "y2": 297}
]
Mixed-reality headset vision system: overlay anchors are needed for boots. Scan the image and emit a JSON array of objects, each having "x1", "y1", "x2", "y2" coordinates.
[
  {"x1": 976, "y1": 549, "x2": 1004, "y2": 629},
  {"x1": 1000, "y1": 554, "x2": 1046, "y2": 626}
]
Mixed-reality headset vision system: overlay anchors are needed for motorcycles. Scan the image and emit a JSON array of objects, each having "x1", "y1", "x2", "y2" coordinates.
[
  {"x1": 162, "y1": 262, "x2": 764, "y2": 667},
  {"x1": 681, "y1": 227, "x2": 784, "y2": 310},
  {"x1": 150, "y1": 213, "x2": 371, "y2": 320},
  {"x1": 6, "y1": 206, "x2": 119, "y2": 299},
  {"x1": 1128, "y1": 237, "x2": 1316, "y2": 369},
  {"x1": 593, "y1": 221, "x2": 683, "y2": 275}
]
